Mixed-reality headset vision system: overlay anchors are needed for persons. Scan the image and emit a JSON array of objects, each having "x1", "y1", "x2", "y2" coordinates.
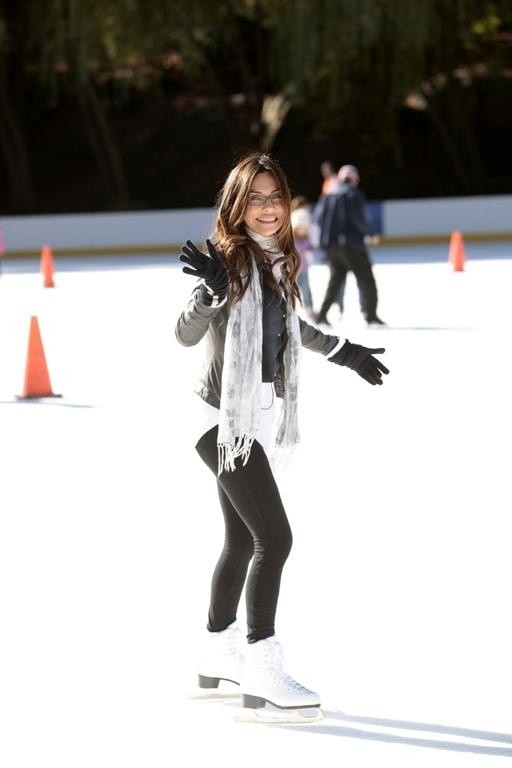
[
  {"x1": 291, "y1": 161, "x2": 384, "y2": 326},
  {"x1": 174, "y1": 154, "x2": 390, "y2": 712}
]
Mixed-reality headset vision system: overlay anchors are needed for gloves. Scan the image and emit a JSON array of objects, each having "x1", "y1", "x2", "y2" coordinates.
[
  {"x1": 179, "y1": 239, "x2": 228, "y2": 308},
  {"x1": 324, "y1": 336, "x2": 389, "y2": 385}
]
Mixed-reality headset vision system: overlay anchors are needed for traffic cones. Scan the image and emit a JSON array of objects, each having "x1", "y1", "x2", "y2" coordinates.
[{"x1": 17, "y1": 316, "x2": 61, "y2": 400}]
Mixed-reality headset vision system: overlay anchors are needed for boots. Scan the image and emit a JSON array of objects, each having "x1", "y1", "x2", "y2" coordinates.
[{"x1": 195, "y1": 619, "x2": 321, "y2": 709}]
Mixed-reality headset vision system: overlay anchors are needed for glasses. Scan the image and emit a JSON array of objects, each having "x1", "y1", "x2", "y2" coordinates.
[{"x1": 246, "y1": 193, "x2": 283, "y2": 206}]
